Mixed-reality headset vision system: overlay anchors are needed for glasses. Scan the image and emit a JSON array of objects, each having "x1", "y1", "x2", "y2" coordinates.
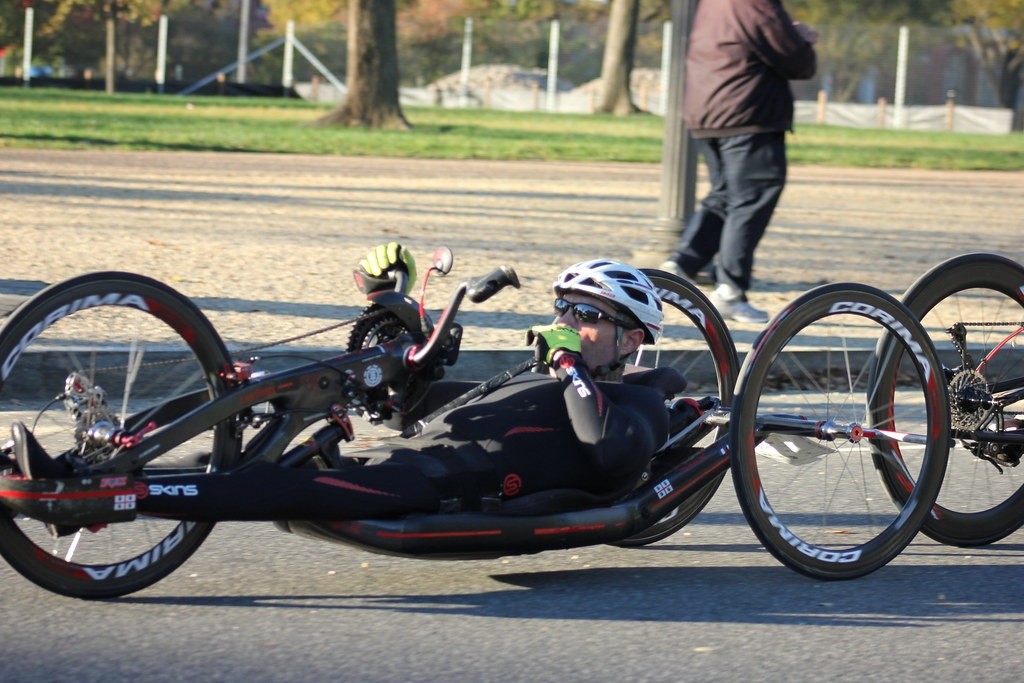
[{"x1": 553, "y1": 298, "x2": 635, "y2": 331}]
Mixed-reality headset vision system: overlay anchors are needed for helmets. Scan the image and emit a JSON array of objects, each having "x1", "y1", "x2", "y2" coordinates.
[{"x1": 553, "y1": 258, "x2": 664, "y2": 345}]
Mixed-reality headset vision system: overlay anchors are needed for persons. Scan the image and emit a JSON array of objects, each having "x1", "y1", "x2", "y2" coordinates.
[
  {"x1": 657, "y1": 0, "x2": 819, "y2": 323},
  {"x1": 999, "y1": 37, "x2": 1024, "y2": 132},
  {"x1": 10, "y1": 241, "x2": 666, "y2": 539}
]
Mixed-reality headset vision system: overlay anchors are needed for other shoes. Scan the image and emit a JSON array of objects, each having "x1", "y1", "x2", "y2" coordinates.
[
  {"x1": 660, "y1": 261, "x2": 696, "y2": 286},
  {"x1": 709, "y1": 290, "x2": 769, "y2": 323},
  {"x1": 12, "y1": 420, "x2": 82, "y2": 539}
]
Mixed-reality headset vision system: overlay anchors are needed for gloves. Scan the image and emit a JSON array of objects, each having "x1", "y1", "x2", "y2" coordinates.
[
  {"x1": 361, "y1": 242, "x2": 417, "y2": 297},
  {"x1": 525, "y1": 324, "x2": 581, "y2": 365}
]
